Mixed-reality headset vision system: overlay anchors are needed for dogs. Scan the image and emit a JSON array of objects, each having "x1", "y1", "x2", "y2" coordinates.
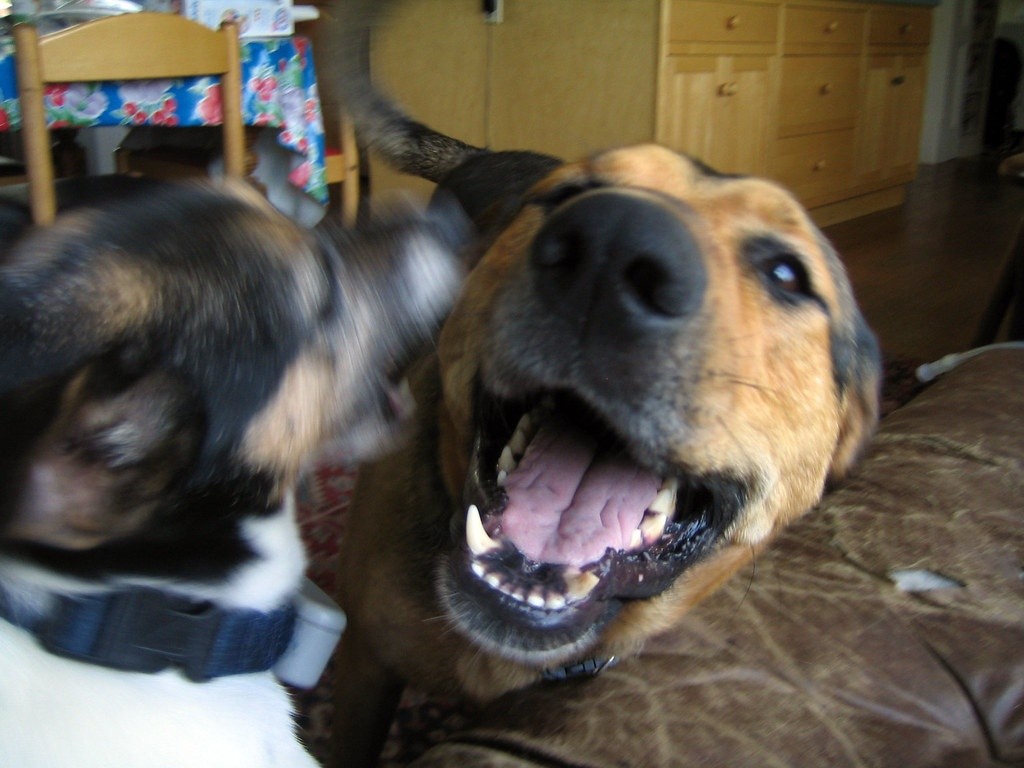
[{"x1": 0, "y1": 0, "x2": 888, "y2": 768}]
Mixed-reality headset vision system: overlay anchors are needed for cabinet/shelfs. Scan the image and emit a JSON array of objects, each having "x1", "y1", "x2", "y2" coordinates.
[{"x1": 369, "y1": 0, "x2": 936, "y2": 229}]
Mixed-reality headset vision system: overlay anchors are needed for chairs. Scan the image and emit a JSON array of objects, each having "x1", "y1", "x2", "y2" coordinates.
[{"x1": 13, "y1": 12, "x2": 245, "y2": 224}]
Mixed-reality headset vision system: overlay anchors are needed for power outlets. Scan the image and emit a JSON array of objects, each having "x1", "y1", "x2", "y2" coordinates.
[{"x1": 486, "y1": 0, "x2": 503, "y2": 25}]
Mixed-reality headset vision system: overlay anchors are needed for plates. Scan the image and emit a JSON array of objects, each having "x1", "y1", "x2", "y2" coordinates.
[{"x1": 38, "y1": 0, "x2": 144, "y2": 26}]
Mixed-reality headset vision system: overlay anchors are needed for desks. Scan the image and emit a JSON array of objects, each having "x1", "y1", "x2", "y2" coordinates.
[{"x1": 0, "y1": 36, "x2": 329, "y2": 229}]
